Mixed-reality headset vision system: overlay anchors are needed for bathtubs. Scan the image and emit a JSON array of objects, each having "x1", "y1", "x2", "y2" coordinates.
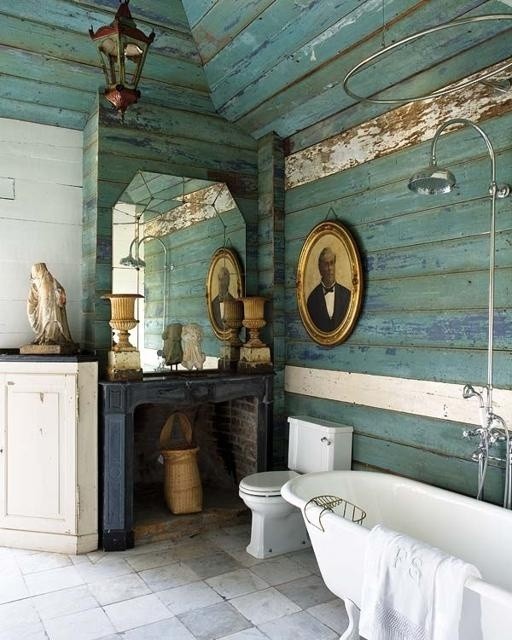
[{"x1": 279, "y1": 469, "x2": 512, "y2": 640}]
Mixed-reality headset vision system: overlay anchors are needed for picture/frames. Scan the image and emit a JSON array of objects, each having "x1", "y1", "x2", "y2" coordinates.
[
  {"x1": 206, "y1": 246, "x2": 243, "y2": 341},
  {"x1": 296, "y1": 222, "x2": 363, "y2": 345}
]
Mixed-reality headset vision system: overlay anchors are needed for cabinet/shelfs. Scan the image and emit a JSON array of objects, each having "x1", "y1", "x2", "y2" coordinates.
[{"x1": 0, "y1": 374, "x2": 78, "y2": 535}]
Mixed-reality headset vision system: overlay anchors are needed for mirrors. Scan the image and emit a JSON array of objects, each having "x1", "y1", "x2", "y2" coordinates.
[{"x1": 112, "y1": 171, "x2": 246, "y2": 374}]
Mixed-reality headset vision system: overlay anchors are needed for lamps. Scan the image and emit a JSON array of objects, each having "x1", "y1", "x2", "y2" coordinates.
[
  {"x1": 242, "y1": 297, "x2": 267, "y2": 348},
  {"x1": 88, "y1": 1, "x2": 156, "y2": 124},
  {"x1": 99, "y1": 292, "x2": 145, "y2": 351}
]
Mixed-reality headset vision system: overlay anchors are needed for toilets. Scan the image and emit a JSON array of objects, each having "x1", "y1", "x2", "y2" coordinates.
[{"x1": 238, "y1": 416, "x2": 354, "y2": 560}]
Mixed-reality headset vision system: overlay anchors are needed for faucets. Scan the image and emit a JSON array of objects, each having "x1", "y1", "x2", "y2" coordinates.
[{"x1": 471, "y1": 449, "x2": 485, "y2": 462}]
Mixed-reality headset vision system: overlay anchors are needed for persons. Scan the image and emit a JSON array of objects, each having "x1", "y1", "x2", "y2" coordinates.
[
  {"x1": 212, "y1": 267, "x2": 235, "y2": 330},
  {"x1": 306, "y1": 247, "x2": 351, "y2": 333}
]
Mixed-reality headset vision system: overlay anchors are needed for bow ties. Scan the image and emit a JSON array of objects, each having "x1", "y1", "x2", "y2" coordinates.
[{"x1": 321, "y1": 283, "x2": 336, "y2": 295}]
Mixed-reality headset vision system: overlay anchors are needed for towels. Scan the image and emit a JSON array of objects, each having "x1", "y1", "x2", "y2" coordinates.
[{"x1": 358, "y1": 524, "x2": 482, "y2": 639}]
